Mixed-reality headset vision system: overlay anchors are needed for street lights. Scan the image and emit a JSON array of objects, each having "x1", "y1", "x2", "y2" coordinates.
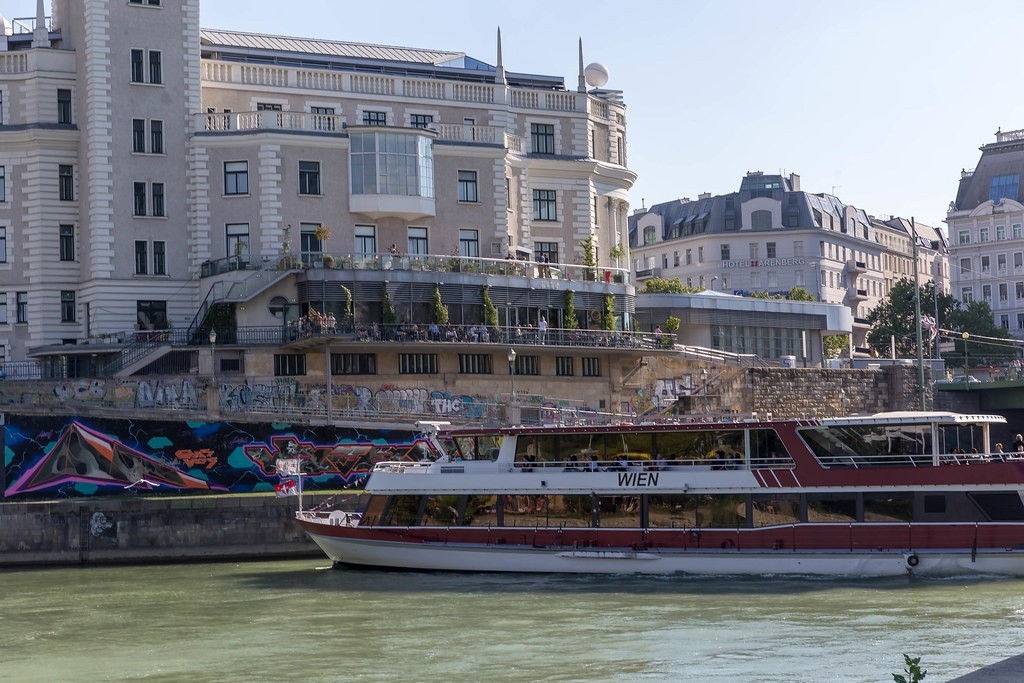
[
  {"x1": 701, "y1": 369, "x2": 707, "y2": 415},
  {"x1": 928, "y1": 282, "x2": 940, "y2": 359},
  {"x1": 207, "y1": 327, "x2": 220, "y2": 419},
  {"x1": 506, "y1": 348, "x2": 521, "y2": 427},
  {"x1": 711, "y1": 276, "x2": 718, "y2": 290},
  {"x1": 962, "y1": 332, "x2": 969, "y2": 382}
]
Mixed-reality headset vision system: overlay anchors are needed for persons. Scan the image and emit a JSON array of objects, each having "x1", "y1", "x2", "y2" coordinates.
[
  {"x1": 287, "y1": 311, "x2": 664, "y2": 349},
  {"x1": 522, "y1": 451, "x2": 744, "y2": 471},
  {"x1": 389, "y1": 244, "x2": 398, "y2": 257},
  {"x1": 1009, "y1": 360, "x2": 1024, "y2": 379},
  {"x1": 505, "y1": 253, "x2": 526, "y2": 276},
  {"x1": 939, "y1": 434, "x2": 1024, "y2": 465},
  {"x1": 538, "y1": 253, "x2": 552, "y2": 278}
]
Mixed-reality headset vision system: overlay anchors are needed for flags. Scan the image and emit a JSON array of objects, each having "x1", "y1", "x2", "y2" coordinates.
[
  {"x1": 276, "y1": 459, "x2": 298, "y2": 474},
  {"x1": 275, "y1": 480, "x2": 298, "y2": 498}
]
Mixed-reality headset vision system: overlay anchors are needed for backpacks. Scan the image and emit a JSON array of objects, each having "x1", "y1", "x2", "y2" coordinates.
[
  {"x1": 1018, "y1": 445, "x2": 1023, "y2": 451},
  {"x1": 657, "y1": 330, "x2": 662, "y2": 338}
]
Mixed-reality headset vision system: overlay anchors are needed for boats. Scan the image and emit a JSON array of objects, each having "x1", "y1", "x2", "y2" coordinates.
[{"x1": 295, "y1": 411, "x2": 1024, "y2": 579}]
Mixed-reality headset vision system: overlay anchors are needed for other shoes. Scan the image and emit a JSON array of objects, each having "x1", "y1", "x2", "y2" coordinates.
[{"x1": 542, "y1": 343, "x2": 544, "y2": 345}]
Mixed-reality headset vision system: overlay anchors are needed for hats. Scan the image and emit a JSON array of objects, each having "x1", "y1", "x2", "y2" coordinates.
[
  {"x1": 329, "y1": 313, "x2": 333, "y2": 315},
  {"x1": 960, "y1": 449, "x2": 965, "y2": 453},
  {"x1": 590, "y1": 456, "x2": 597, "y2": 461}
]
